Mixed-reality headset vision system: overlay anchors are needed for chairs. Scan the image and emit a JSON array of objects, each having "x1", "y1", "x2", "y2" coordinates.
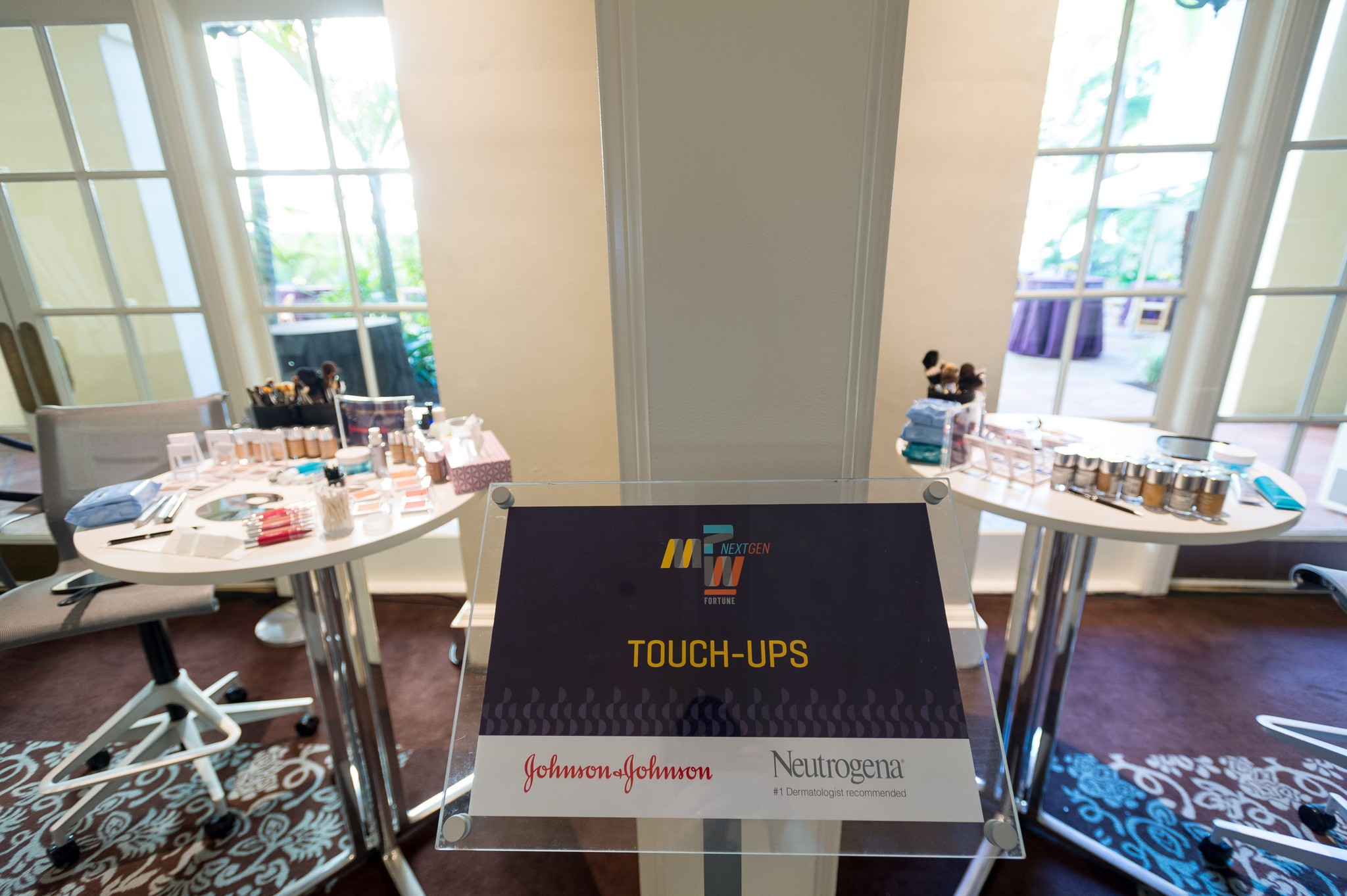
[{"x1": 0, "y1": 391, "x2": 324, "y2": 870}]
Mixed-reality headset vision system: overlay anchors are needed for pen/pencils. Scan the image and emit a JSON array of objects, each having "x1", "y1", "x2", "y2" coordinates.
[
  {"x1": 109, "y1": 529, "x2": 175, "y2": 547},
  {"x1": 1067, "y1": 486, "x2": 1145, "y2": 519}
]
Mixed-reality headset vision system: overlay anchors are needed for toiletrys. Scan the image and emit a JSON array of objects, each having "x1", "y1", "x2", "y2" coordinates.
[
  {"x1": 1247, "y1": 468, "x2": 1310, "y2": 512},
  {"x1": 1224, "y1": 469, "x2": 1261, "y2": 506}
]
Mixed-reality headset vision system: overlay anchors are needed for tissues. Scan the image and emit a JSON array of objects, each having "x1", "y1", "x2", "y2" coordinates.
[{"x1": 443, "y1": 414, "x2": 514, "y2": 496}]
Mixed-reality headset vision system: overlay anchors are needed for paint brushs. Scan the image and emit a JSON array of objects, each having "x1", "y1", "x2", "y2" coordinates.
[{"x1": 246, "y1": 360, "x2": 343, "y2": 406}]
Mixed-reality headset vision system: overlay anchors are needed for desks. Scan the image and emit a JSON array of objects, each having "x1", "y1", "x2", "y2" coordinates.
[
  {"x1": 891, "y1": 411, "x2": 1307, "y2": 896},
  {"x1": 81, "y1": 444, "x2": 481, "y2": 893}
]
